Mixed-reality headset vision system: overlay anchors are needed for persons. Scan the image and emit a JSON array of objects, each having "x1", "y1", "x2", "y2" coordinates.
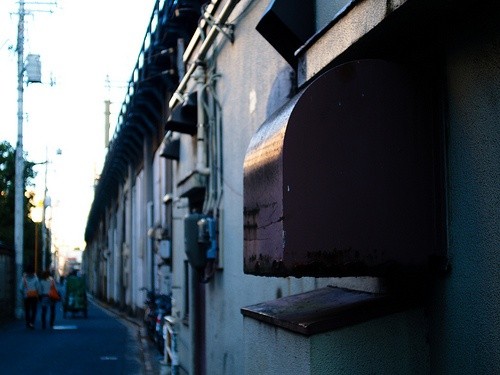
[
  {"x1": 20, "y1": 267, "x2": 39, "y2": 329},
  {"x1": 65, "y1": 269, "x2": 81, "y2": 319},
  {"x1": 37, "y1": 270, "x2": 61, "y2": 333}
]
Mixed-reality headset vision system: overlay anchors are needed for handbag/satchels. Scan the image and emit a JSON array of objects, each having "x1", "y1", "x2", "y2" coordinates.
[
  {"x1": 48, "y1": 280, "x2": 60, "y2": 301},
  {"x1": 27, "y1": 288, "x2": 39, "y2": 302}
]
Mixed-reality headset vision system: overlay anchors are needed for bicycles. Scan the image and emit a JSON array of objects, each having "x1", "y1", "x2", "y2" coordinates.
[
  {"x1": 153, "y1": 293, "x2": 172, "y2": 353},
  {"x1": 141, "y1": 287, "x2": 161, "y2": 341}
]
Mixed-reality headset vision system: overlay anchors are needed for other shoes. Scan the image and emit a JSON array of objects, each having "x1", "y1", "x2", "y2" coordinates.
[{"x1": 27, "y1": 323, "x2": 35, "y2": 329}]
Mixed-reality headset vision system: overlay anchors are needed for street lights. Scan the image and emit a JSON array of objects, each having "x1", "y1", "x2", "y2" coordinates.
[{"x1": 41, "y1": 145, "x2": 63, "y2": 271}]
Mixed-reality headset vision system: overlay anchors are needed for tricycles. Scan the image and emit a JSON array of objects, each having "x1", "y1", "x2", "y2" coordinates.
[{"x1": 61, "y1": 277, "x2": 88, "y2": 320}]
